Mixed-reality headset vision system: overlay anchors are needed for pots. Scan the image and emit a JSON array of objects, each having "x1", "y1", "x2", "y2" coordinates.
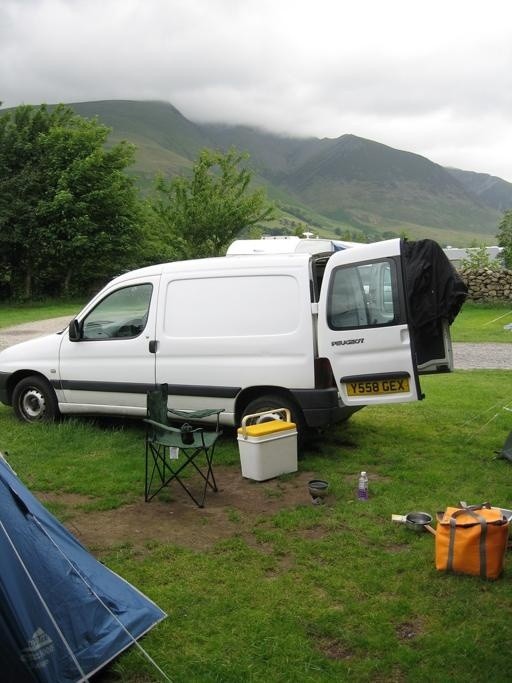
[{"x1": 405, "y1": 511, "x2": 439, "y2": 537}]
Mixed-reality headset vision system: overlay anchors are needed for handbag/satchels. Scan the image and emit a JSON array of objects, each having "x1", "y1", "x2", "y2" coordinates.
[{"x1": 435, "y1": 500, "x2": 510, "y2": 582}]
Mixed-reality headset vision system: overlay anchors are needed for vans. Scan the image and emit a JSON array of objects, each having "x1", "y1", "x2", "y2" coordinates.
[
  {"x1": 226, "y1": 233, "x2": 394, "y2": 319},
  {"x1": 1, "y1": 236, "x2": 466, "y2": 445}
]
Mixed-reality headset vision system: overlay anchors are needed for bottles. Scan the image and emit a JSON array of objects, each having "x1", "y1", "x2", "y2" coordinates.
[
  {"x1": 182, "y1": 423, "x2": 195, "y2": 446},
  {"x1": 358, "y1": 471, "x2": 369, "y2": 501}
]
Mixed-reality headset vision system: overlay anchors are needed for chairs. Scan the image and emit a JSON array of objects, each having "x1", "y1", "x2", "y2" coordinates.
[{"x1": 142, "y1": 381, "x2": 226, "y2": 509}]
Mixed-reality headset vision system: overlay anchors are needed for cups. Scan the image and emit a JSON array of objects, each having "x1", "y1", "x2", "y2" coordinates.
[
  {"x1": 169, "y1": 446, "x2": 179, "y2": 460},
  {"x1": 308, "y1": 479, "x2": 330, "y2": 504}
]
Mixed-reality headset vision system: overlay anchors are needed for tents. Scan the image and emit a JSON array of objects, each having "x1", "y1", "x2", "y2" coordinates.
[{"x1": 0, "y1": 450, "x2": 168, "y2": 683}]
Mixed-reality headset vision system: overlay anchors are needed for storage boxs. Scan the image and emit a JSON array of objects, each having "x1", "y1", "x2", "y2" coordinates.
[{"x1": 235, "y1": 406, "x2": 299, "y2": 482}]
